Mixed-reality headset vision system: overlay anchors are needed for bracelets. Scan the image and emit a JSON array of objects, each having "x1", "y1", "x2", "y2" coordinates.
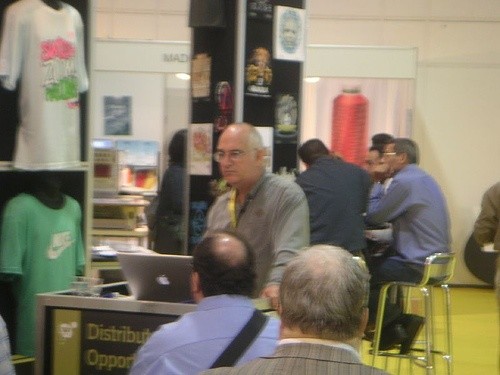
[{"x1": 373, "y1": 180, "x2": 384, "y2": 186}]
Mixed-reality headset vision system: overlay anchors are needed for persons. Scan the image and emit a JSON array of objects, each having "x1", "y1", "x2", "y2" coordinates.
[
  {"x1": 198, "y1": 245, "x2": 397, "y2": 375},
  {"x1": 130, "y1": 232, "x2": 282, "y2": 375},
  {"x1": 364, "y1": 138, "x2": 454, "y2": 356},
  {"x1": 0, "y1": 173, "x2": 89, "y2": 359},
  {"x1": 152, "y1": 128, "x2": 188, "y2": 254},
  {"x1": 200, "y1": 122, "x2": 308, "y2": 316},
  {"x1": 363, "y1": 133, "x2": 407, "y2": 340},
  {"x1": 465, "y1": 180, "x2": 500, "y2": 288},
  {"x1": 295, "y1": 138, "x2": 375, "y2": 257},
  {"x1": 0, "y1": 0, "x2": 89, "y2": 170}
]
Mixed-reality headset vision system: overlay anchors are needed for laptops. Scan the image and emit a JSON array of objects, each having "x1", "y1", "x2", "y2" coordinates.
[{"x1": 116, "y1": 252, "x2": 193, "y2": 302}]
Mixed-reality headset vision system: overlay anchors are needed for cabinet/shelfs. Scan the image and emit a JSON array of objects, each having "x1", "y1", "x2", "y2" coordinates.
[{"x1": 0, "y1": 0, "x2": 94, "y2": 375}]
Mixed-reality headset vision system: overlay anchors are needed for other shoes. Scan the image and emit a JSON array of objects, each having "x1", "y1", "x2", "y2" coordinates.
[
  {"x1": 399, "y1": 314, "x2": 426, "y2": 355},
  {"x1": 371, "y1": 324, "x2": 408, "y2": 350}
]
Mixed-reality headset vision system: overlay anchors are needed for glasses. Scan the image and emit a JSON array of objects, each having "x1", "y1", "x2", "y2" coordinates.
[{"x1": 213, "y1": 149, "x2": 259, "y2": 162}]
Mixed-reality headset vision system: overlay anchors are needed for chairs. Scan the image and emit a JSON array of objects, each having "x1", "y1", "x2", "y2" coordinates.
[{"x1": 370, "y1": 251, "x2": 454, "y2": 375}]
